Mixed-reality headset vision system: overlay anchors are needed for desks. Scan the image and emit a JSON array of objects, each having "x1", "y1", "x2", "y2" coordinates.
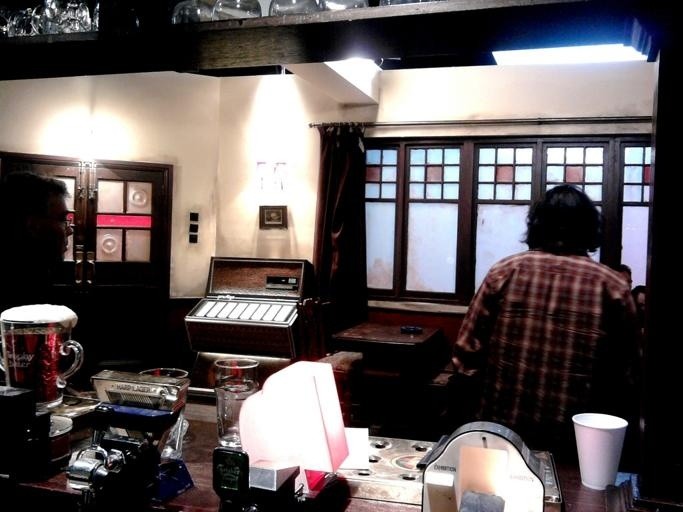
[
  {"x1": 0, "y1": 402, "x2": 628, "y2": 512},
  {"x1": 333, "y1": 323, "x2": 440, "y2": 379}
]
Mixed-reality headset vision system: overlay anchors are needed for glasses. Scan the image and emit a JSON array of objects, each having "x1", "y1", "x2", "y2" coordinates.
[{"x1": 51, "y1": 216, "x2": 72, "y2": 227}]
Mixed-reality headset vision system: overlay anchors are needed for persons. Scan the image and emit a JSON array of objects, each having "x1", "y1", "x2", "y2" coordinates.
[
  {"x1": 612, "y1": 265, "x2": 633, "y2": 292},
  {"x1": 631, "y1": 286, "x2": 645, "y2": 356},
  {"x1": 451, "y1": 182, "x2": 643, "y2": 458},
  {"x1": 0, "y1": 172, "x2": 74, "y2": 310}
]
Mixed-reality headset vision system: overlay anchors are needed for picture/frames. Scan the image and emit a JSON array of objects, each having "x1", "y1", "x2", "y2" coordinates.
[{"x1": 259, "y1": 205, "x2": 288, "y2": 230}]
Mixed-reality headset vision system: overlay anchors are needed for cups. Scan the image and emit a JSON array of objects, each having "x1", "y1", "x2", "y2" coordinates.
[
  {"x1": 138, "y1": 367, "x2": 189, "y2": 379},
  {"x1": 572, "y1": 413, "x2": 628, "y2": 490},
  {"x1": 214, "y1": 359, "x2": 260, "y2": 448}
]
